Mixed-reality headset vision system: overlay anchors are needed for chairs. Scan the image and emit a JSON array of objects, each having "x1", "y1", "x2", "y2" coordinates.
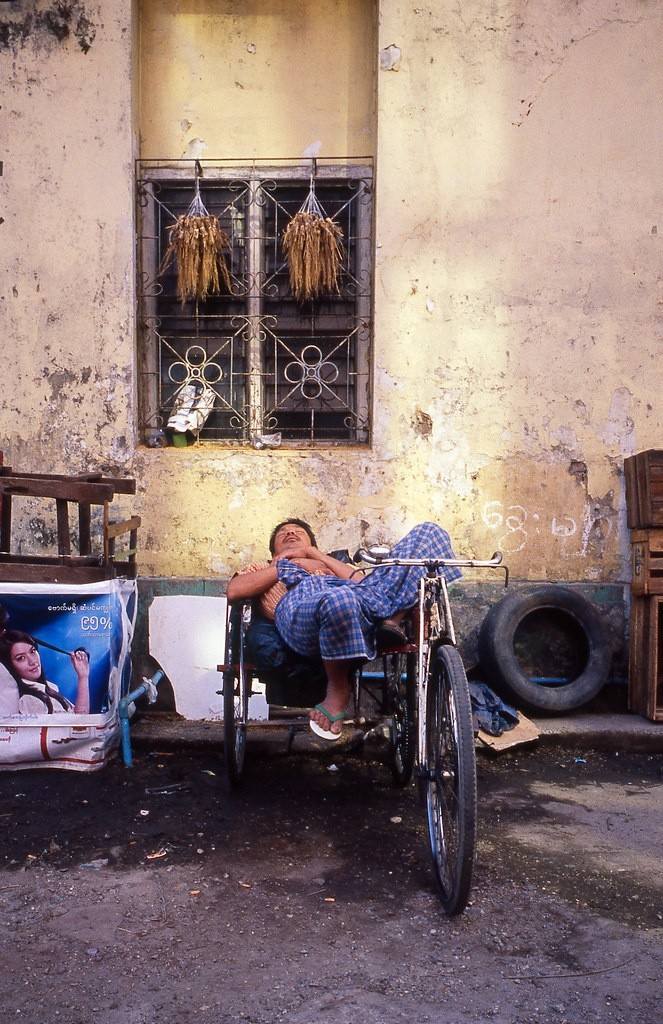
[{"x1": 226, "y1": 549, "x2": 367, "y2": 726}]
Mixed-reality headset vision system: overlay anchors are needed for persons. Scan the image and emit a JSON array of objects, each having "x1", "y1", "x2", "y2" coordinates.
[
  {"x1": 225, "y1": 515, "x2": 462, "y2": 743},
  {"x1": 0, "y1": 629, "x2": 91, "y2": 714}
]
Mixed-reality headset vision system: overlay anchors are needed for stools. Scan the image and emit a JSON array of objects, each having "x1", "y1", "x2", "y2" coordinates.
[{"x1": 0, "y1": 467, "x2": 141, "y2": 584}]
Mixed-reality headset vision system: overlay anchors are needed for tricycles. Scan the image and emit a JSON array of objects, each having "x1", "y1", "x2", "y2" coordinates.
[{"x1": 215, "y1": 541, "x2": 509, "y2": 915}]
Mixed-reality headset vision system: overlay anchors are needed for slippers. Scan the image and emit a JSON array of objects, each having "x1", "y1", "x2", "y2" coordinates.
[
  {"x1": 374, "y1": 626, "x2": 406, "y2": 649},
  {"x1": 309, "y1": 703, "x2": 346, "y2": 741}
]
